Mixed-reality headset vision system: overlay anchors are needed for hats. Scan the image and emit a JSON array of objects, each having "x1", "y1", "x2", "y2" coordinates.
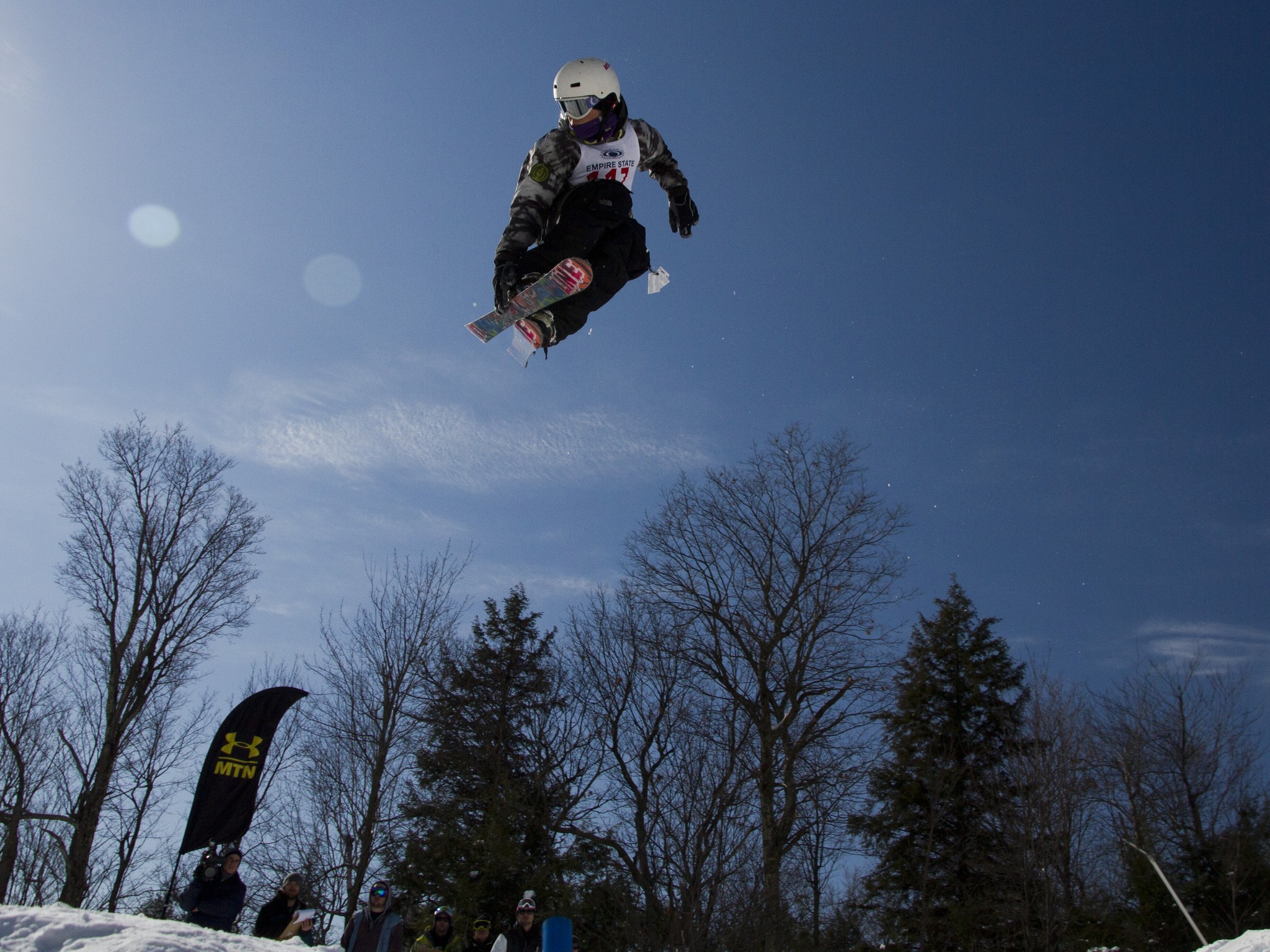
[
  {"x1": 476, "y1": 913, "x2": 492, "y2": 928},
  {"x1": 222, "y1": 847, "x2": 242, "y2": 863},
  {"x1": 281, "y1": 872, "x2": 303, "y2": 888}
]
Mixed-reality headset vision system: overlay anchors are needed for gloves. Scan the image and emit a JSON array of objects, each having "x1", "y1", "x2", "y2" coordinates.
[
  {"x1": 667, "y1": 186, "x2": 699, "y2": 238},
  {"x1": 493, "y1": 260, "x2": 515, "y2": 315}
]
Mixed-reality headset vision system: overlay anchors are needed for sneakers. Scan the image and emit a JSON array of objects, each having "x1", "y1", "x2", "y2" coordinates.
[
  {"x1": 528, "y1": 309, "x2": 556, "y2": 361},
  {"x1": 507, "y1": 272, "x2": 542, "y2": 301}
]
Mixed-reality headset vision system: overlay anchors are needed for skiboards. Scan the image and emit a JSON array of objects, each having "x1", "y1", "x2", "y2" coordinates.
[{"x1": 464, "y1": 256, "x2": 595, "y2": 369}]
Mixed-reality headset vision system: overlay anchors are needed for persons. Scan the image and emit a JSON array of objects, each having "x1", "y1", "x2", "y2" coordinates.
[
  {"x1": 493, "y1": 55, "x2": 700, "y2": 351},
  {"x1": 177, "y1": 848, "x2": 544, "y2": 952}
]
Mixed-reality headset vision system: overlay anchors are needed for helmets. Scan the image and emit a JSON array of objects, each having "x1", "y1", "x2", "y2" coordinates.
[
  {"x1": 554, "y1": 57, "x2": 621, "y2": 105},
  {"x1": 434, "y1": 906, "x2": 453, "y2": 920},
  {"x1": 517, "y1": 898, "x2": 536, "y2": 911}
]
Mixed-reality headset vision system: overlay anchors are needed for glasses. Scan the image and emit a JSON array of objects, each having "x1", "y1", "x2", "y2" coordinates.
[
  {"x1": 555, "y1": 96, "x2": 592, "y2": 120},
  {"x1": 434, "y1": 916, "x2": 450, "y2": 923},
  {"x1": 370, "y1": 887, "x2": 388, "y2": 898},
  {"x1": 472, "y1": 920, "x2": 490, "y2": 930},
  {"x1": 517, "y1": 908, "x2": 535, "y2": 915},
  {"x1": 518, "y1": 898, "x2": 535, "y2": 908}
]
